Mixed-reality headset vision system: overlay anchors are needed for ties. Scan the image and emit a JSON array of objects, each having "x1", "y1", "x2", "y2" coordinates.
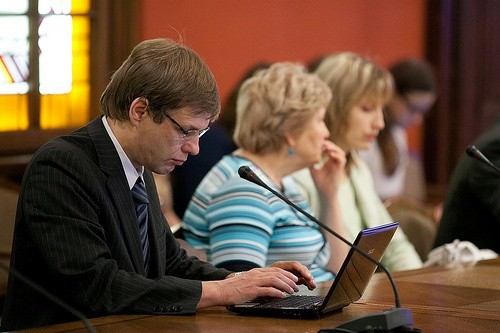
[{"x1": 131, "y1": 176, "x2": 151, "y2": 278}]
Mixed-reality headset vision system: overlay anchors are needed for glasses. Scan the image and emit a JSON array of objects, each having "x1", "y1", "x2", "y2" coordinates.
[
  {"x1": 127, "y1": 96, "x2": 211, "y2": 140},
  {"x1": 400, "y1": 92, "x2": 428, "y2": 115}
]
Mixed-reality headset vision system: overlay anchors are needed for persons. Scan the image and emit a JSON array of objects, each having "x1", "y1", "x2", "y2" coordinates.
[
  {"x1": 183, "y1": 62, "x2": 347, "y2": 282},
  {"x1": 315, "y1": 53, "x2": 423, "y2": 270},
  {"x1": 153, "y1": 57, "x2": 440, "y2": 240},
  {"x1": 2, "y1": 39, "x2": 315, "y2": 328},
  {"x1": 432, "y1": 121, "x2": 499, "y2": 254}
]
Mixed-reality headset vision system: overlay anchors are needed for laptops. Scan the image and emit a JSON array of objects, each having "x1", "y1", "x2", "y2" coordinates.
[{"x1": 226, "y1": 221, "x2": 399, "y2": 320}]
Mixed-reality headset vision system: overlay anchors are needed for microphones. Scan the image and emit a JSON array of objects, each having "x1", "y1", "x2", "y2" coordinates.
[
  {"x1": 238, "y1": 165, "x2": 400, "y2": 309},
  {"x1": 466, "y1": 146, "x2": 500, "y2": 173},
  {"x1": 0, "y1": 263, "x2": 94, "y2": 333}
]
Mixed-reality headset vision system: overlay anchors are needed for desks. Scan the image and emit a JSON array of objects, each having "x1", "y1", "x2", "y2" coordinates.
[{"x1": 13, "y1": 254, "x2": 500, "y2": 333}]
